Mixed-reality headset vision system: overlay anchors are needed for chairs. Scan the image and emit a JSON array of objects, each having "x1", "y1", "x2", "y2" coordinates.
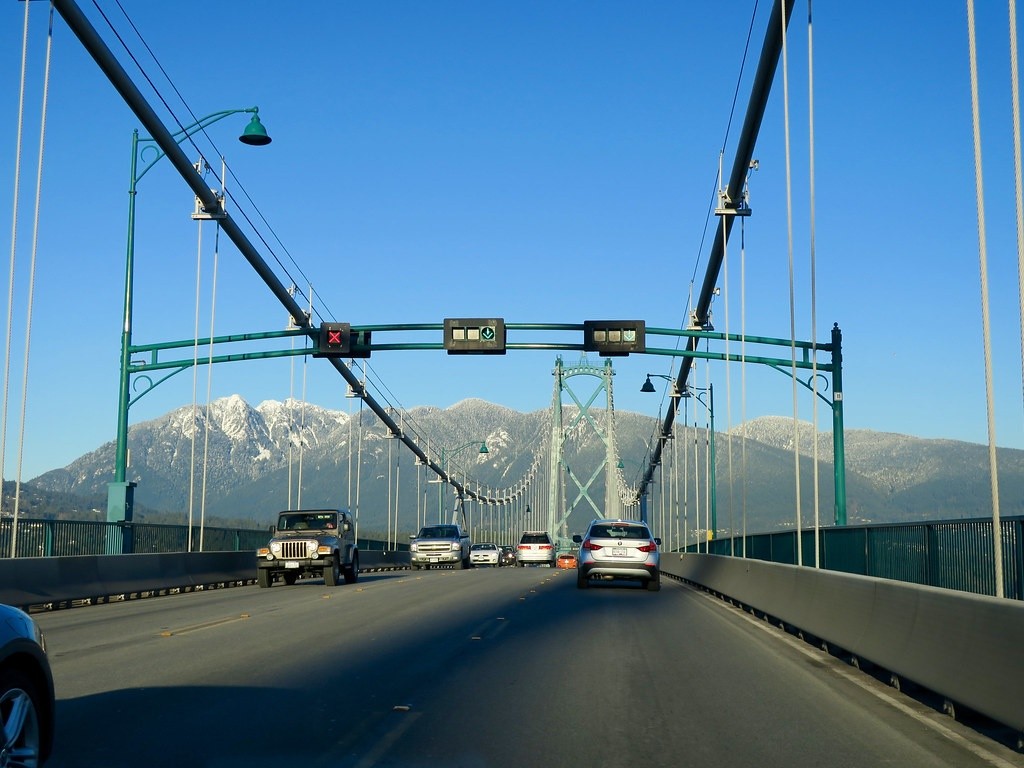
[
  {"x1": 627, "y1": 533, "x2": 639, "y2": 537},
  {"x1": 294, "y1": 522, "x2": 308, "y2": 529}
]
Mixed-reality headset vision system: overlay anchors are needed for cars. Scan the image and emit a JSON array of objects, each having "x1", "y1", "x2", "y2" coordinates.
[
  {"x1": 498, "y1": 545, "x2": 516, "y2": 567},
  {"x1": 556, "y1": 554, "x2": 577, "y2": 569},
  {"x1": 469, "y1": 543, "x2": 503, "y2": 566},
  {"x1": 0, "y1": 601, "x2": 56, "y2": 768}
]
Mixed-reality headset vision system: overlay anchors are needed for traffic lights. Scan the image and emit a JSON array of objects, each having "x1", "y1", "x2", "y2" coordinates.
[
  {"x1": 443, "y1": 318, "x2": 506, "y2": 351},
  {"x1": 584, "y1": 320, "x2": 646, "y2": 352},
  {"x1": 318, "y1": 320, "x2": 352, "y2": 354}
]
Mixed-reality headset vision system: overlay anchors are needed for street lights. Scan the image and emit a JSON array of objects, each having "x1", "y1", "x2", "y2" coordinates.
[
  {"x1": 439, "y1": 439, "x2": 490, "y2": 524},
  {"x1": 641, "y1": 373, "x2": 717, "y2": 541}
]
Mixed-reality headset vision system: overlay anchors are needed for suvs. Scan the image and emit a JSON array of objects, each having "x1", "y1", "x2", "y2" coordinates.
[
  {"x1": 409, "y1": 525, "x2": 472, "y2": 570},
  {"x1": 572, "y1": 519, "x2": 663, "y2": 592},
  {"x1": 256, "y1": 509, "x2": 360, "y2": 587}
]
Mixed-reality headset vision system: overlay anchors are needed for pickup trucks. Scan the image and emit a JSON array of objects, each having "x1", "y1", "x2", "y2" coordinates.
[{"x1": 515, "y1": 531, "x2": 558, "y2": 568}]
